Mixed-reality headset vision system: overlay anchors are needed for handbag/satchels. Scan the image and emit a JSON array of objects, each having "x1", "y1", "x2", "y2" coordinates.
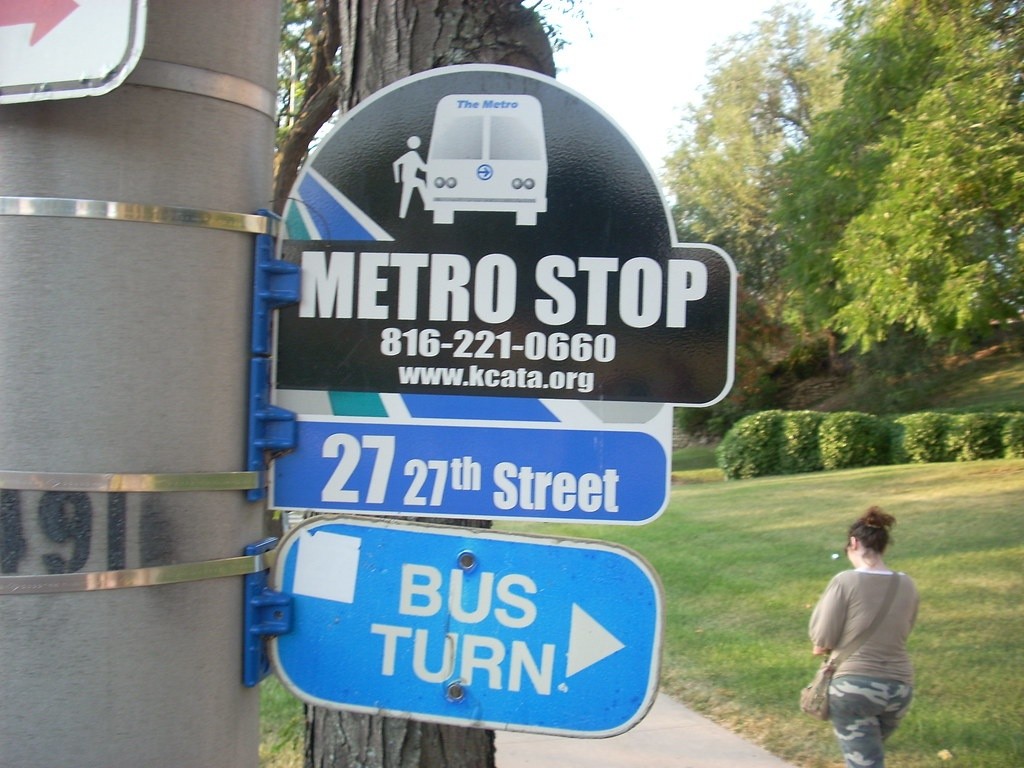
[{"x1": 800, "y1": 662, "x2": 834, "y2": 720}]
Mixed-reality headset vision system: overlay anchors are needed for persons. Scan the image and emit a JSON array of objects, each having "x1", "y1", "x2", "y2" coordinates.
[{"x1": 807, "y1": 506, "x2": 921, "y2": 768}]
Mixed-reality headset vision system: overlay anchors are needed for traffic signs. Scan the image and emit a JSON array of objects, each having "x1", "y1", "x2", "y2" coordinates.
[
  {"x1": 231, "y1": 239, "x2": 741, "y2": 527},
  {"x1": 260, "y1": 515, "x2": 666, "y2": 743}
]
